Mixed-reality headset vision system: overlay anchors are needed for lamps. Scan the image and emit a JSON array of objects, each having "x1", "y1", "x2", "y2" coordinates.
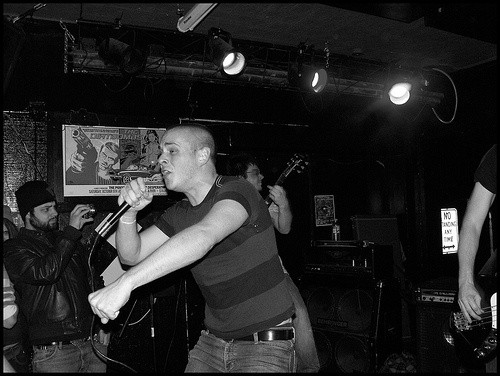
[
  {"x1": 202, "y1": 26, "x2": 246, "y2": 77},
  {"x1": 286, "y1": 43, "x2": 329, "y2": 94},
  {"x1": 382, "y1": 67, "x2": 413, "y2": 106},
  {"x1": 99, "y1": 20, "x2": 147, "y2": 78}
]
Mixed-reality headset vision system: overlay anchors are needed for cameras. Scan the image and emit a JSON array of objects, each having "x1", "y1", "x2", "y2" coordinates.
[{"x1": 82, "y1": 205, "x2": 96, "y2": 219}]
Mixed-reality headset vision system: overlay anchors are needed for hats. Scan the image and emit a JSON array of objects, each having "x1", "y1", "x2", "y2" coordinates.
[{"x1": 14, "y1": 180, "x2": 57, "y2": 220}]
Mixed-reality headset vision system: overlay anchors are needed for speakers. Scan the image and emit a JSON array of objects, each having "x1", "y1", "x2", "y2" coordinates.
[
  {"x1": 299, "y1": 274, "x2": 402, "y2": 376},
  {"x1": 414, "y1": 276, "x2": 500, "y2": 376}
]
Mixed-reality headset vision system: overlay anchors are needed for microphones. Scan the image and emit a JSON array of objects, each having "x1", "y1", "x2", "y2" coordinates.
[{"x1": 97, "y1": 201, "x2": 132, "y2": 237}]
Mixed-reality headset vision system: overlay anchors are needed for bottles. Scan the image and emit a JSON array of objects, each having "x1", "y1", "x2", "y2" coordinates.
[{"x1": 332, "y1": 219, "x2": 340, "y2": 241}]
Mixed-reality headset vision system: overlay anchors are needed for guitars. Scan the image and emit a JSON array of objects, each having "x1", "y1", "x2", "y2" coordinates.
[
  {"x1": 265, "y1": 151, "x2": 310, "y2": 206},
  {"x1": 448, "y1": 277, "x2": 500, "y2": 367}
]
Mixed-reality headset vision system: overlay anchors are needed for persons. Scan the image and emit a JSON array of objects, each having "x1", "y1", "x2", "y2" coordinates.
[
  {"x1": 457, "y1": 143, "x2": 500, "y2": 324},
  {"x1": 227, "y1": 146, "x2": 320, "y2": 373},
  {"x1": 3, "y1": 179, "x2": 110, "y2": 373},
  {"x1": 88, "y1": 124, "x2": 297, "y2": 373}
]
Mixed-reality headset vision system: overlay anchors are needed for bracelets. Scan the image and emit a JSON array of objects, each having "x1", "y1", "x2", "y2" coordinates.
[{"x1": 119, "y1": 216, "x2": 136, "y2": 225}]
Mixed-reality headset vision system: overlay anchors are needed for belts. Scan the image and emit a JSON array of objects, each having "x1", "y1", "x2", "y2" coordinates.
[{"x1": 237, "y1": 324, "x2": 296, "y2": 344}]
